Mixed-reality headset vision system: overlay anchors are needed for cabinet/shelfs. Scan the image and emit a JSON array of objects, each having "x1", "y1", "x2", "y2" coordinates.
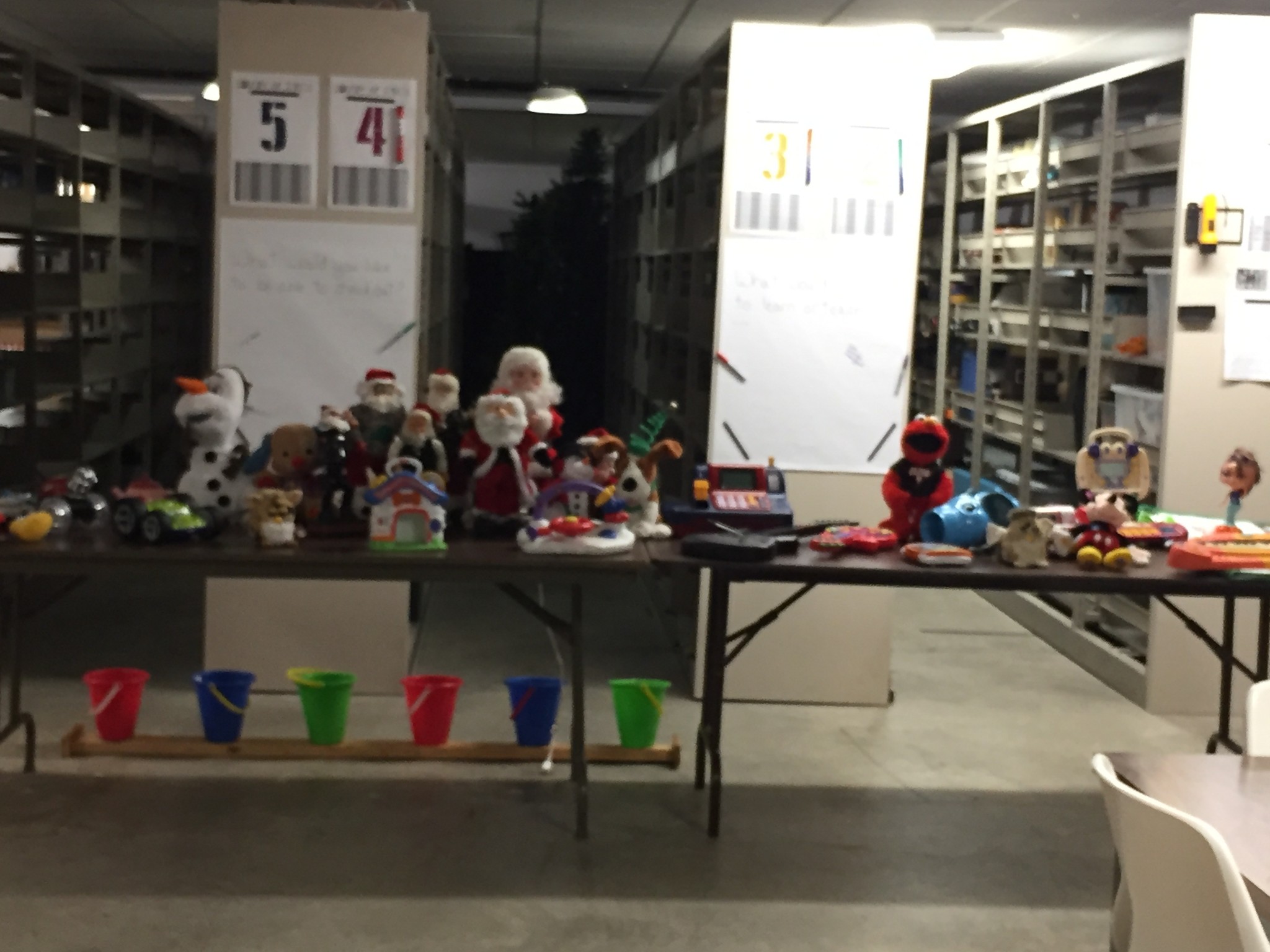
[{"x1": 0, "y1": 0, "x2": 1270, "y2": 716}]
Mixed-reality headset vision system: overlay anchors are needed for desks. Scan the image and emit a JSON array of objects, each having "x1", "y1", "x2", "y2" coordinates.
[
  {"x1": 1, "y1": 522, "x2": 650, "y2": 838},
  {"x1": 645, "y1": 536, "x2": 1269, "y2": 837},
  {"x1": 1096, "y1": 750, "x2": 1269, "y2": 936}
]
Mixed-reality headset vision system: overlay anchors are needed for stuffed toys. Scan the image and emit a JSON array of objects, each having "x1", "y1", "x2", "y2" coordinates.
[
  {"x1": 167, "y1": 342, "x2": 681, "y2": 541},
  {"x1": 1049, "y1": 486, "x2": 1151, "y2": 569},
  {"x1": 878, "y1": 411, "x2": 952, "y2": 543},
  {"x1": 1074, "y1": 427, "x2": 1151, "y2": 516},
  {"x1": 999, "y1": 509, "x2": 1056, "y2": 569},
  {"x1": 1220, "y1": 450, "x2": 1261, "y2": 533}
]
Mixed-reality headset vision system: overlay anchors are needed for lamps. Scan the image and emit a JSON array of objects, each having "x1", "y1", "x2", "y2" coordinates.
[{"x1": 523, "y1": 84, "x2": 589, "y2": 114}]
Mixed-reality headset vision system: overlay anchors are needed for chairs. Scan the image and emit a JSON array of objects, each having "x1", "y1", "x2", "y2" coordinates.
[{"x1": 1090, "y1": 754, "x2": 1270, "y2": 952}]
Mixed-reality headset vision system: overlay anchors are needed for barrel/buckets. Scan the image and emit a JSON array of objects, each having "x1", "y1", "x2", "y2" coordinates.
[
  {"x1": 193, "y1": 668, "x2": 256, "y2": 743},
  {"x1": 84, "y1": 667, "x2": 149, "y2": 742},
  {"x1": 401, "y1": 674, "x2": 462, "y2": 746},
  {"x1": 611, "y1": 679, "x2": 671, "y2": 750},
  {"x1": 503, "y1": 676, "x2": 563, "y2": 747},
  {"x1": 286, "y1": 666, "x2": 357, "y2": 745}
]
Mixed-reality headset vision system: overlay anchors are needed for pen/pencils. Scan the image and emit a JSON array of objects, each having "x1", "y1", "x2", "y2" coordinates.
[
  {"x1": 868, "y1": 425, "x2": 896, "y2": 463},
  {"x1": 723, "y1": 422, "x2": 751, "y2": 460},
  {"x1": 397, "y1": 104, "x2": 403, "y2": 165},
  {"x1": 714, "y1": 354, "x2": 745, "y2": 382}
]
